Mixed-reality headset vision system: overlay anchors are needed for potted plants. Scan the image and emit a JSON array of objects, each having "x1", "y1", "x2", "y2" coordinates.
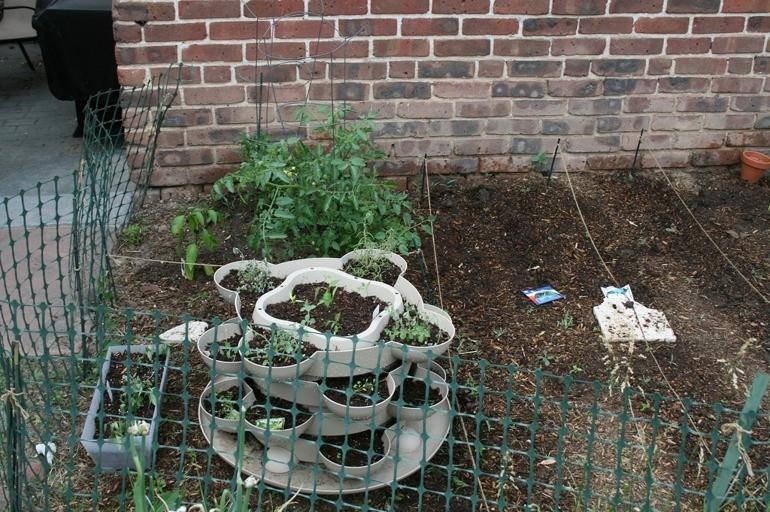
[{"x1": 80, "y1": 342, "x2": 176, "y2": 474}]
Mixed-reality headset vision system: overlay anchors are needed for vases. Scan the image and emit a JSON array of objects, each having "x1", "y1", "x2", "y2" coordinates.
[{"x1": 738, "y1": 150, "x2": 770, "y2": 184}]
